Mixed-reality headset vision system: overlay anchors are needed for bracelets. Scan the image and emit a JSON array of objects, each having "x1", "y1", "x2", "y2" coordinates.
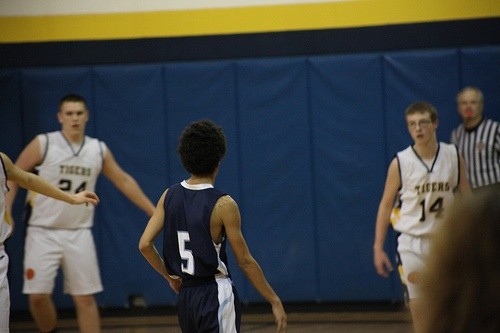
[{"x1": 164, "y1": 275, "x2": 171, "y2": 280}]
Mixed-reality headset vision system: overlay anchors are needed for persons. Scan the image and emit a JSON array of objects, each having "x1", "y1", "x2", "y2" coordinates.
[
  {"x1": 5, "y1": 94, "x2": 158, "y2": 333},
  {"x1": 137, "y1": 118, "x2": 290, "y2": 332},
  {"x1": 372, "y1": 100, "x2": 478, "y2": 333},
  {"x1": 1, "y1": 153, "x2": 102, "y2": 333},
  {"x1": 446, "y1": 85, "x2": 500, "y2": 188}
]
